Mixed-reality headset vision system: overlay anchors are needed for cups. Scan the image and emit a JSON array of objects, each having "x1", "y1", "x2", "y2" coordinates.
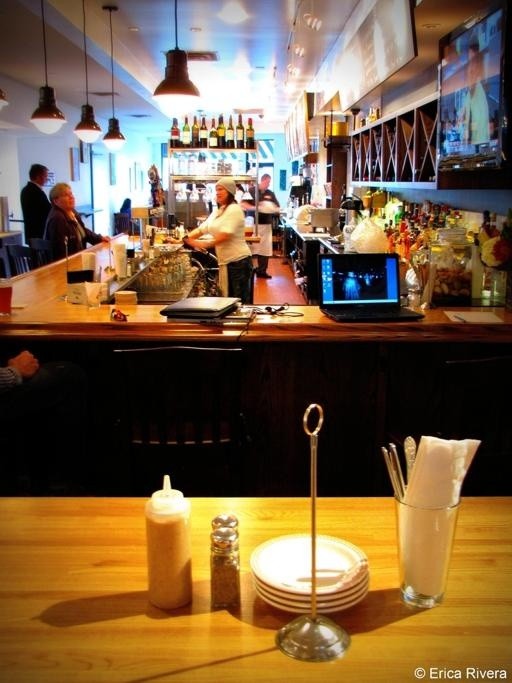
[
  {"x1": 127, "y1": 250, "x2": 192, "y2": 297},
  {"x1": 391, "y1": 493, "x2": 460, "y2": 610},
  {"x1": 0, "y1": 278, "x2": 14, "y2": 315}
]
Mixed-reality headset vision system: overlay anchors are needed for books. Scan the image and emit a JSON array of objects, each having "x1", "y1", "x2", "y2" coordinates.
[{"x1": 159, "y1": 298, "x2": 256, "y2": 324}]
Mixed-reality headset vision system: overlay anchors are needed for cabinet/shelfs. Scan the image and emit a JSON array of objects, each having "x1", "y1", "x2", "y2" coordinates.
[
  {"x1": 168, "y1": 141, "x2": 261, "y2": 243},
  {"x1": 350, "y1": 91, "x2": 438, "y2": 189}
]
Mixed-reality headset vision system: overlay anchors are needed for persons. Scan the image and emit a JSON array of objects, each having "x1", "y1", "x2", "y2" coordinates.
[
  {"x1": 234, "y1": 184, "x2": 246, "y2": 206},
  {"x1": 452, "y1": 36, "x2": 491, "y2": 145},
  {"x1": 241, "y1": 174, "x2": 281, "y2": 279},
  {"x1": 119, "y1": 199, "x2": 133, "y2": 217},
  {"x1": 20, "y1": 163, "x2": 51, "y2": 245},
  {"x1": 42, "y1": 182, "x2": 110, "y2": 264},
  {"x1": 161, "y1": 175, "x2": 255, "y2": 306},
  {"x1": 0, "y1": 350, "x2": 40, "y2": 386}
]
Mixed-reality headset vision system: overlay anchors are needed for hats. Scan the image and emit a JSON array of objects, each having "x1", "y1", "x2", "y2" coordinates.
[{"x1": 215, "y1": 176, "x2": 237, "y2": 197}]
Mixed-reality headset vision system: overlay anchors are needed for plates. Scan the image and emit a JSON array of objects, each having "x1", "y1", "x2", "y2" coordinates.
[{"x1": 248, "y1": 542, "x2": 370, "y2": 613}]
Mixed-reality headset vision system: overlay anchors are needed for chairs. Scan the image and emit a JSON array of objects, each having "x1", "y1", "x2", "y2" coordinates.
[
  {"x1": 111, "y1": 345, "x2": 258, "y2": 459},
  {"x1": 29, "y1": 235, "x2": 68, "y2": 268},
  {"x1": 2, "y1": 244, "x2": 38, "y2": 278}
]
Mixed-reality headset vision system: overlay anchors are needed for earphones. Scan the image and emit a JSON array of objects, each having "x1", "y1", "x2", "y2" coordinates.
[{"x1": 264, "y1": 303, "x2": 303, "y2": 317}]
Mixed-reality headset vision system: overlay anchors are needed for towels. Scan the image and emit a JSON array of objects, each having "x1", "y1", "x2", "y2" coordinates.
[{"x1": 399, "y1": 434, "x2": 482, "y2": 597}]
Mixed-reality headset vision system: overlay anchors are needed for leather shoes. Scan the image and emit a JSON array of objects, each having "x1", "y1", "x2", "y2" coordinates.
[{"x1": 256, "y1": 271, "x2": 273, "y2": 279}]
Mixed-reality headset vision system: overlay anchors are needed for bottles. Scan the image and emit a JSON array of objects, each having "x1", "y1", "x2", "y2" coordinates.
[
  {"x1": 209, "y1": 117, "x2": 219, "y2": 148},
  {"x1": 200, "y1": 117, "x2": 208, "y2": 148},
  {"x1": 236, "y1": 115, "x2": 244, "y2": 149},
  {"x1": 182, "y1": 117, "x2": 191, "y2": 148},
  {"x1": 209, "y1": 517, "x2": 246, "y2": 610},
  {"x1": 170, "y1": 118, "x2": 180, "y2": 148},
  {"x1": 382, "y1": 191, "x2": 474, "y2": 246},
  {"x1": 343, "y1": 210, "x2": 358, "y2": 252},
  {"x1": 192, "y1": 116, "x2": 199, "y2": 148},
  {"x1": 428, "y1": 228, "x2": 472, "y2": 308},
  {"x1": 189, "y1": 183, "x2": 199, "y2": 203},
  {"x1": 176, "y1": 183, "x2": 188, "y2": 203},
  {"x1": 478, "y1": 209, "x2": 500, "y2": 248},
  {"x1": 368, "y1": 207, "x2": 379, "y2": 224},
  {"x1": 187, "y1": 154, "x2": 194, "y2": 175},
  {"x1": 143, "y1": 472, "x2": 198, "y2": 610},
  {"x1": 227, "y1": 117, "x2": 234, "y2": 149},
  {"x1": 246, "y1": 118, "x2": 254, "y2": 149},
  {"x1": 216, "y1": 115, "x2": 226, "y2": 148}
]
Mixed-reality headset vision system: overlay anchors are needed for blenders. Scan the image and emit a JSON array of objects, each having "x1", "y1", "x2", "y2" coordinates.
[{"x1": 340, "y1": 196, "x2": 365, "y2": 234}]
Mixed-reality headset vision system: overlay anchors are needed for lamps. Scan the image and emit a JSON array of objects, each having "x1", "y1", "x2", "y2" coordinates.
[
  {"x1": 153, "y1": 2, "x2": 200, "y2": 115},
  {"x1": 302, "y1": 13, "x2": 322, "y2": 32},
  {"x1": 30, "y1": 1, "x2": 67, "y2": 134},
  {"x1": 102, "y1": 6, "x2": 126, "y2": 151},
  {"x1": 74, "y1": 1, "x2": 102, "y2": 143}
]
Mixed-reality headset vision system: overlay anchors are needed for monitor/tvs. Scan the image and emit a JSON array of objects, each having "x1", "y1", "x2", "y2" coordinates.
[{"x1": 436, "y1": 1, "x2": 511, "y2": 189}]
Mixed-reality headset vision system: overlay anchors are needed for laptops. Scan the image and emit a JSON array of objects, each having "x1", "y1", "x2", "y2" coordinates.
[{"x1": 317, "y1": 253, "x2": 425, "y2": 322}]
